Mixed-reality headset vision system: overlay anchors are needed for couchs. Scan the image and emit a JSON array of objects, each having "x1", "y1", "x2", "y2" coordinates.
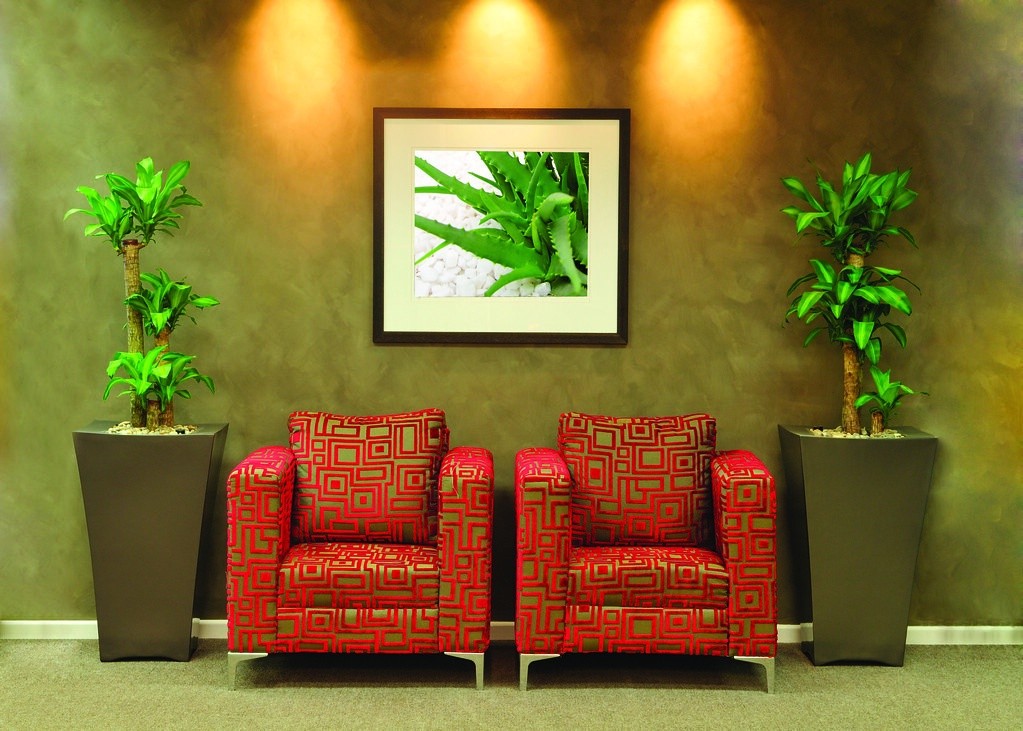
[
  {"x1": 226, "y1": 408, "x2": 495, "y2": 692},
  {"x1": 514, "y1": 412, "x2": 777, "y2": 695}
]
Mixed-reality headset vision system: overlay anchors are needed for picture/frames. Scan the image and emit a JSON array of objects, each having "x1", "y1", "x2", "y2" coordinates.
[{"x1": 372, "y1": 107, "x2": 629, "y2": 347}]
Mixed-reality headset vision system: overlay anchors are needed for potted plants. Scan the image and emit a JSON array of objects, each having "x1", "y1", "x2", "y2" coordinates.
[
  {"x1": 778, "y1": 149, "x2": 941, "y2": 667},
  {"x1": 61, "y1": 155, "x2": 230, "y2": 663}
]
